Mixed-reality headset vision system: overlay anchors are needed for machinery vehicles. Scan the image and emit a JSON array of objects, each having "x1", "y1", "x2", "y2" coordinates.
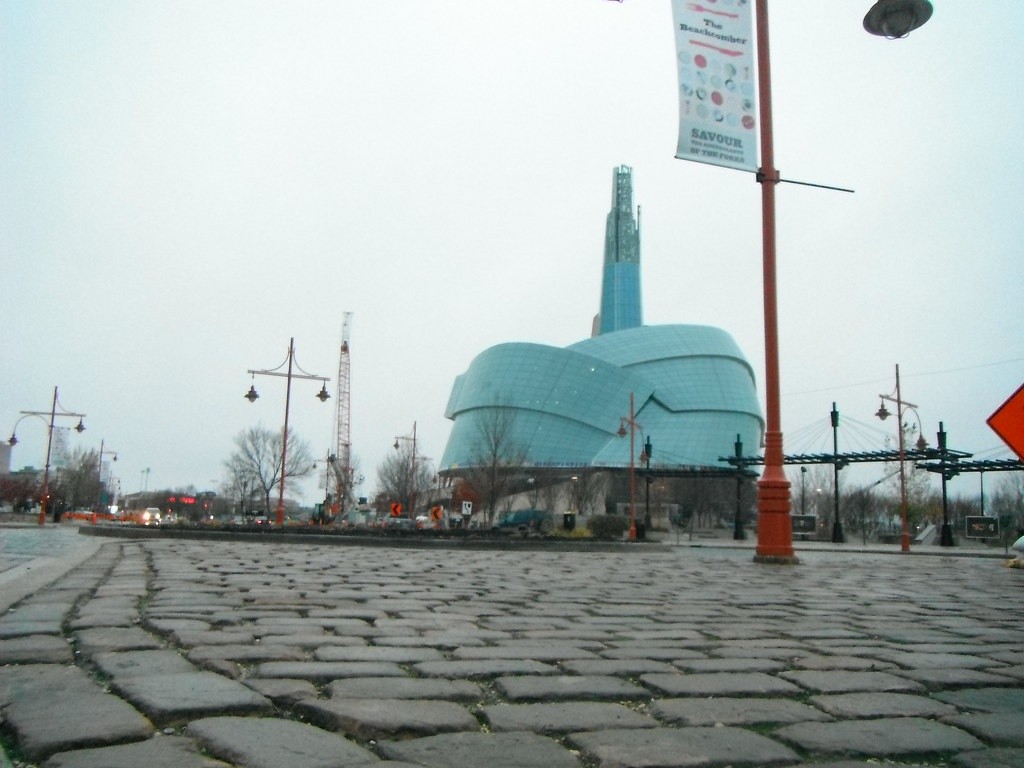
[{"x1": 327, "y1": 454, "x2": 371, "y2": 512}]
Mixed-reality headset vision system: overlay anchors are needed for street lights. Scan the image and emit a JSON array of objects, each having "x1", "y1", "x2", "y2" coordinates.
[
  {"x1": 874, "y1": 395, "x2": 930, "y2": 551},
  {"x1": 393, "y1": 437, "x2": 416, "y2": 467},
  {"x1": 801, "y1": 467, "x2": 806, "y2": 540},
  {"x1": 617, "y1": 417, "x2": 648, "y2": 539},
  {"x1": 753, "y1": 1, "x2": 933, "y2": 565},
  {"x1": 979, "y1": 466, "x2": 985, "y2": 543},
  {"x1": 244, "y1": 370, "x2": 331, "y2": 526},
  {"x1": 99, "y1": 451, "x2": 118, "y2": 472},
  {"x1": 312, "y1": 449, "x2": 329, "y2": 498},
  {"x1": 8, "y1": 413, "x2": 86, "y2": 526}
]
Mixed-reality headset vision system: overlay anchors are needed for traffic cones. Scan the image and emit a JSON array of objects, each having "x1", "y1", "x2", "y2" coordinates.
[{"x1": 92, "y1": 512, "x2": 97, "y2": 525}]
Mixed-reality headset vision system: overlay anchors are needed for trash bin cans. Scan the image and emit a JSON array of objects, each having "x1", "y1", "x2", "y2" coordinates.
[
  {"x1": 563, "y1": 513, "x2": 576, "y2": 529},
  {"x1": 635, "y1": 519, "x2": 645, "y2": 538}
]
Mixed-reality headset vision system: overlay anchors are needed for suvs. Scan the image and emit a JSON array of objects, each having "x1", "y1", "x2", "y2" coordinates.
[{"x1": 491, "y1": 510, "x2": 554, "y2": 535}]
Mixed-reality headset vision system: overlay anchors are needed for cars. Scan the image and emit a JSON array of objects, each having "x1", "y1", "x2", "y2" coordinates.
[
  {"x1": 234, "y1": 511, "x2": 268, "y2": 525},
  {"x1": 383, "y1": 513, "x2": 416, "y2": 529},
  {"x1": 143, "y1": 508, "x2": 160, "y2": 522}
]
